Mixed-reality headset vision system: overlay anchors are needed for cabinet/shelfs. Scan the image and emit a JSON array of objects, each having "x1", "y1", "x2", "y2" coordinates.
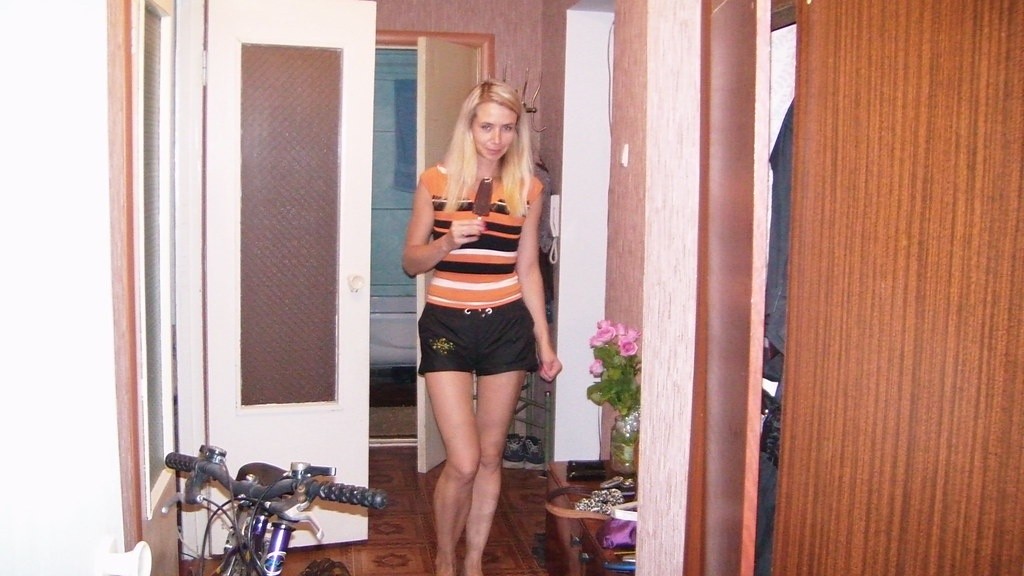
[{"x1": 545, "y1": 459, "x2": 636, "y2": 576}]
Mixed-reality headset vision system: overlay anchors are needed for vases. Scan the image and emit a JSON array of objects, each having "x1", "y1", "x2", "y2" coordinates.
[{"x1": 609, "y1": 416, "x2": 640, "y2": 472}]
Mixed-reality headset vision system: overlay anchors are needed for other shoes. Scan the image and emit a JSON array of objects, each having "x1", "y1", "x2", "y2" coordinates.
[
  {"x1": 502, "y1": 433, "x2": 525, "y2": 469},
  {"x1": 524, "y1": 434, "x2": 545, "y2": 470}
]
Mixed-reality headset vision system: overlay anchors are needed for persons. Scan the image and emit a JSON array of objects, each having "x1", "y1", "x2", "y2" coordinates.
[{"x1": 401, "y1": 79, "x2": 562, "y2": 576}]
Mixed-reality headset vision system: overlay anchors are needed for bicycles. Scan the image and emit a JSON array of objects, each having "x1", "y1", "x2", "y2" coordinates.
[{"x1": 162, "y1": 445, "x2": 387, "y2": 576}]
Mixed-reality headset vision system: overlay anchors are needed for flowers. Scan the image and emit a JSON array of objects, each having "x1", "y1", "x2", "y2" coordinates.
[{"x1": 586, "y1": 319, "x2": 641, "y2": 415}]
